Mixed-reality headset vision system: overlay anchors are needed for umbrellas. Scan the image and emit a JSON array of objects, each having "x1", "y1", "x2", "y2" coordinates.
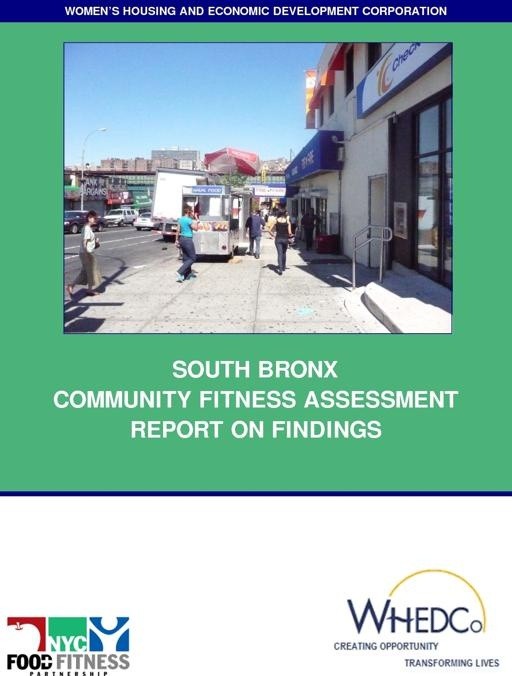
[{"x1": 203, "y1": 146, "x2": 257, "y2": 196}]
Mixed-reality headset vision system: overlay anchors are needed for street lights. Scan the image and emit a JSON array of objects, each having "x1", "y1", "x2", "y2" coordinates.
[{"x1": 79, "y1": 125, "x2": 108, "y2": 210}]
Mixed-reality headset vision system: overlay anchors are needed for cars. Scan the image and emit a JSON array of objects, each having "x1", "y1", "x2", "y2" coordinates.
[
  {"x1": 102, "y1": 208, "x2": 137, "y2": 227},
  {"x1": 132, "y1": 211, "x2": 160, "y2": 230},
  {"x1": 64, "y1": 210, "x2": 107, "y2": 233}
]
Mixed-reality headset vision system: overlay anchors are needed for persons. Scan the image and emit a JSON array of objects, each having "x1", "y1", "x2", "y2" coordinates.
[
  {"x1": 244, "y1": 208, "x2": 266, "y2": 258},
  {"x1": 300, "y1": 207, "x2": 321, "y2": 251},
  {"x1": 283, "y1": 210, "x2": 290, "y2": 225},
  {"x1": 296, "y1": 208, "x2": 307, "y2": 241},
  {"x1": 268, "y1": 208, "x2": 296, "y2": 276},
  {"x1": 171, "y1": 204, "x2": 201, "y2": 283},
  {"x1": 66, "y1": 210, "x2": 100, "y2": 297}
]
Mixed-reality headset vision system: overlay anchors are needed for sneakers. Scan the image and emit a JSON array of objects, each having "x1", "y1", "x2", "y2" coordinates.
[
  {"x1": 86, "y1": 289, "x2": 99, "y2": 296},
  {"x1": 248, "y1": 251, "x2": 254, "y2": 255},
  {"x1": 255, "y1": 254, "x2": 259, "y2": 259},
  {"x1": 66, "y1": 284, "x2": 73, "y2": 297},
  {"x1": 176, "y1": 271, "x2": 184, "y2": 283},
  {"x1": 185, "y1": 272, "x2": 196, "y2": 281},
  {"x1": 278, "y1": 266, "x2": 286, "y2": 274}
]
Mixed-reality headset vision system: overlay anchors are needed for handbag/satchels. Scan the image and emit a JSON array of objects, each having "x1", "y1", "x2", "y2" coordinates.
[{"x1": 94, "y1": 239, "x2": 100, "y2": 249}]
